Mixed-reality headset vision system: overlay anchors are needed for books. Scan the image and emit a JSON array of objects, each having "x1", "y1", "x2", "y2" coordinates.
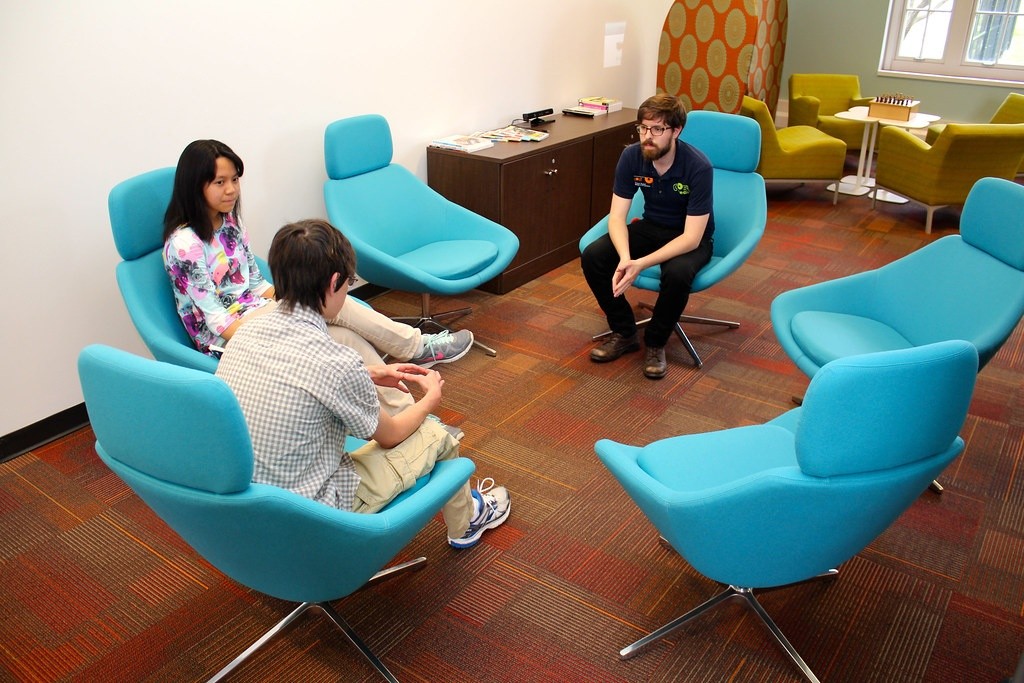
[
  {"x1": 470, "y1": 125, "x2": 549, "y2": 142},
  {"x1": 432, "y1": 135, "x2": 492, "y2": 152},
  {"x1": 563, "y1": 96, "x2": 623, "y2": 116}
]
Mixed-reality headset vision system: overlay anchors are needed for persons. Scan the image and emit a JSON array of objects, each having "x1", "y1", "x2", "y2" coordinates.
[
  {"x1": 214, "y1": 218, "x2": 511, "y2": 548},
  {"x1": 162, "y1": 137, "x2": 474, "y2": 441},
  {"x1": 581, "y1": 92, "x2": 716, "y2": 379}
]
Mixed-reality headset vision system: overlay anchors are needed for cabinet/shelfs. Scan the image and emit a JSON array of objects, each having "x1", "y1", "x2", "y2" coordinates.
[{"x1": 424, "y1": 107, "x2": 641, "y2": 296}]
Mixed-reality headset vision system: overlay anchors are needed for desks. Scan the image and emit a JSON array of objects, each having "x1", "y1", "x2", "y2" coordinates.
[{"x1": 826, "y1": 106, "x2": 941, "y2": 205}]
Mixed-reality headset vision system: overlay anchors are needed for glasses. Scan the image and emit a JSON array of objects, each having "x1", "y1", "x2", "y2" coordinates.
[
  {"x1": 346, "y1": 275, "x2": 358, "y2": 287},
  {"x1": 634, "y1": 122, "x2": 674, "y2": 136}
]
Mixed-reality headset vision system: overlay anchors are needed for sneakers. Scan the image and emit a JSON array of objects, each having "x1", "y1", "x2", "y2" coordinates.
[
  {"x1": 408, "y1": 329, "x2": 473, "y2": 368},
  {"x1": 447, "y1": 477, "x2": 511, "y2": 548},
  {"x1": 426, "y1": 414, "x2": 465, "y2": 440}
]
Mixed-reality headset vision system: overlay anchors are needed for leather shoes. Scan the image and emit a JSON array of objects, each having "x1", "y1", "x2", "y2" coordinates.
[
  {"x1": 643, "y1": 346, "x2": 667, "y2": 376},
  {"x1": 589, "y1": 331, "x2": 641, "y2": 361}
]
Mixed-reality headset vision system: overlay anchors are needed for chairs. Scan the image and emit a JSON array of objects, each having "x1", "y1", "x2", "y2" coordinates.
[
  {"x1": 770, "y1": 176, "x2": 1024, "y2": 493},
  {"x1": 872, "y1": 91, "x2": 1024, "y2": 235},
  {"x1": 75, "y1": 343, "x2": 477, "y2": 683},
  {"x1": 323, "y1": 114, "x2": 520, "y2": 364},
  {"x1": 107, "y1": 166, "x2": 375, "y2": 375},
  {"x1": 739, "y1": 96, "x2": 847, "y2": 205},
  {"x1": 788, "y1": 73, "x2": 906, "y2": 152},
  {"x1": 591, "y1": 341, "x2": 980, "y2": 683},
  {"x1": 578, "y1": 109, "x2": 768, "y2": 370}
]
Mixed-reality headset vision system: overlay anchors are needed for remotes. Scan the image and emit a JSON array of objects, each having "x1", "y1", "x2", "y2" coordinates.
[{"x1": 562, "y1": 109, "x2": 594, "y2": 119}]
